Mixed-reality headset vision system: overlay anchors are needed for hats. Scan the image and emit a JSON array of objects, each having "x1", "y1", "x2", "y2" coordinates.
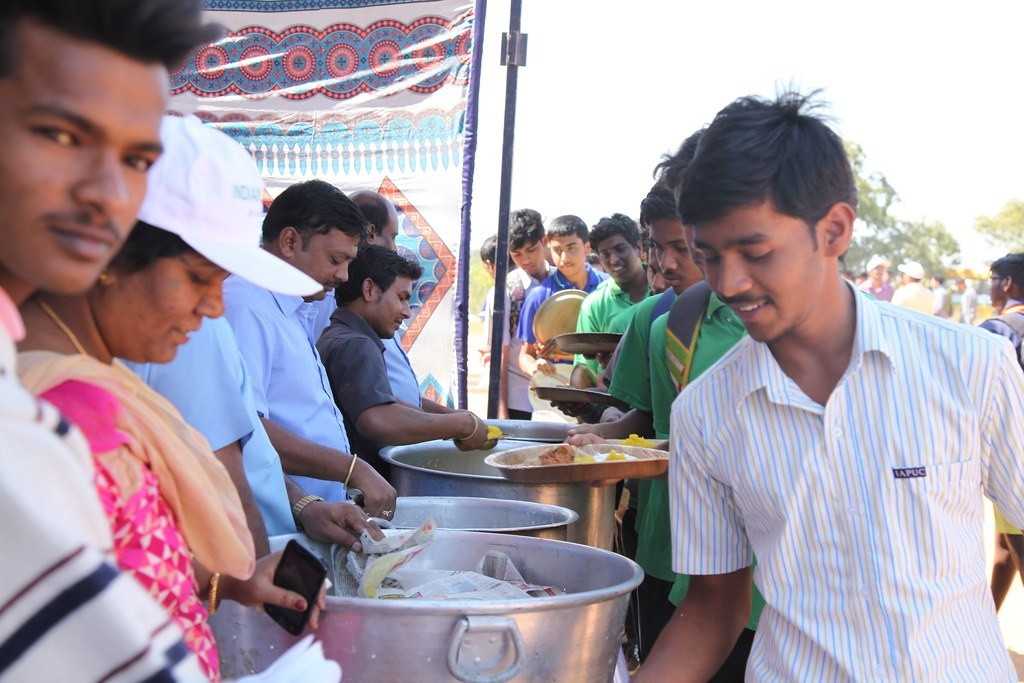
[
  {"x1": 866, "y1": 256, "x2": 891, "y2": 272},
  {"x1": 135, "y1": 113, "x2": 324, "y2": 297},
  {"x1": 898, "y1": 261, "x2": 924, "y2": 279}
]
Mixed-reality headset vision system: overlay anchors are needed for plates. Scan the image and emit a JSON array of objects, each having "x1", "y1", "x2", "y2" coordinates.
[
  {"x1": 571, "y1": 363, "x2": 605, "y2": 423},
  {"x1": 555, "y1": 332, "x2": 623, "y2": 354},
  {"x1": 532, "y1": 289, "x2": 589, "y2": 361},
  {"x1": 529, "y1": 386, "x2": 629, "y2": 406},
  {"x1": 483, "y1": 331, "x2": 670, "y2": 480}
]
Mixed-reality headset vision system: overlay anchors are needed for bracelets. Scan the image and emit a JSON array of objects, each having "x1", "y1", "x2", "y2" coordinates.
[
  {"x1": 208, "y1": 572, "x2": 222, "y2": 616},
  {"x1": 459, "y1": 412, "x2": 478, "y2": 441},
  {"x1": 344, "y1": 453, "x2": 360, "y2": 488},
  {"x1": 292, "y1": 494, "x2": 325, "y2": 522}
]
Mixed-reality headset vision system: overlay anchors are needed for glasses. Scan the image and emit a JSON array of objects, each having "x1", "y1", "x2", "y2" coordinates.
[{"x1": 986, "y1": 275, "x2": 1003, "y2": 285}]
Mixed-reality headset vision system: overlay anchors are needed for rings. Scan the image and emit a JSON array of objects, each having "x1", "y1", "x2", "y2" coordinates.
[
  {"x1": 383, "y1": 511, "x2": 392, "y2": 516},
  {"x1": 366, "y1": 517, "x2": 374, "y2": 523}
]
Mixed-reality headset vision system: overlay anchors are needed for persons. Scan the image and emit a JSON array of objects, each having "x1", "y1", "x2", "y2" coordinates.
[
  {"x1": 980, "y1": 247, "x2": 1023, "y2": 612},
  {"x1": 0, "y1": 1, "x2": 501, "y2": 682},
  {"x1": 481, "y1": 80, "x2": 1023, "y2": 683}
]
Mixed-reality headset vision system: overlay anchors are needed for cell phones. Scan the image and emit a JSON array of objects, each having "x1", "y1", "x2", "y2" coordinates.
[{"x1": 263, "y1": 538, "x2": 328, "y2": 636}]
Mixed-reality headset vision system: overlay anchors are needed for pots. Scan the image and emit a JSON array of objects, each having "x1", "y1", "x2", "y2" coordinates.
[
  {"x1": 377, "y1": 419, "x2": 618, "y2": 553},
  {"x1": 264, "y1": 526, "x2": 645, "y2": 683},
  {"x1": 343, "y1": 495, "x2": 581, "y2": 544}
]
